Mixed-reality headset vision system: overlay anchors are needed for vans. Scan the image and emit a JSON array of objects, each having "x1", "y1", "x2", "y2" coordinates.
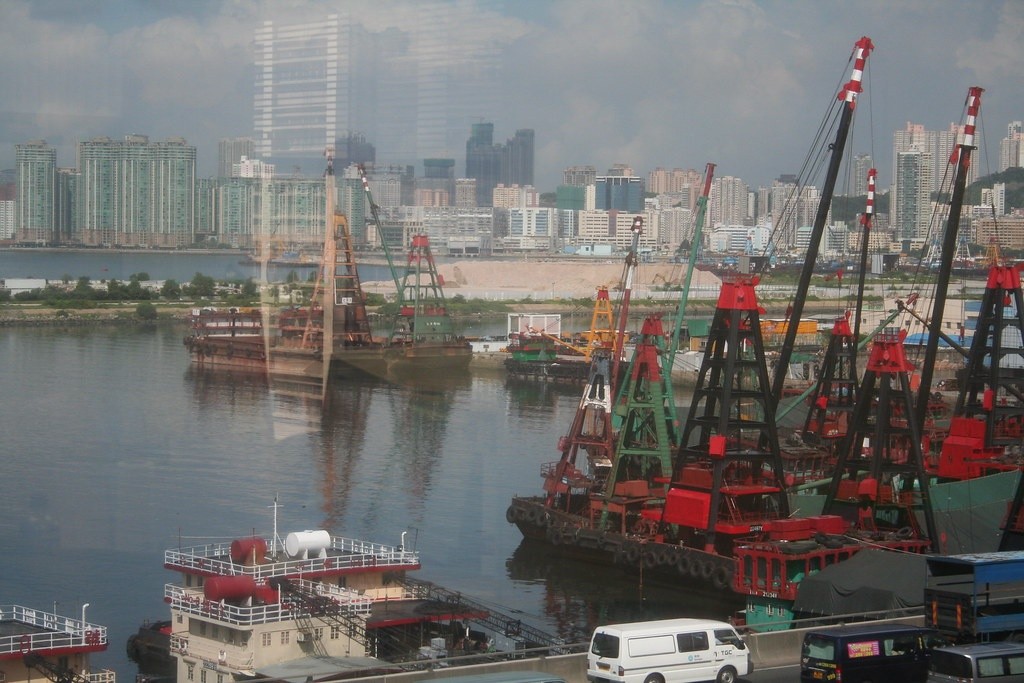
[
  {"x1": 925, "y1": 642, "x2": 1024, "y2": 683},
  {"x1": 799, "y1": 624, "x2": 957, "y2": 683},
  {"x1": 586, "y1": 618, "x2": 755, "y2": 683},
  {"x1": 409, "y1": 668, "x2": 570, "y2": 683}
]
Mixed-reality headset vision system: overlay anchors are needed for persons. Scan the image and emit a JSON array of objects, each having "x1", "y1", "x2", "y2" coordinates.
[{"x1": 714, "y1": 631, "x2": 731, "y2": 644}]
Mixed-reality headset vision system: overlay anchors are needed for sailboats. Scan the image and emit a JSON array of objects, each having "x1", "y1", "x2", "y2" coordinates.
[
  {"x1": 505, "y1": 34, "x2": 1024, "y2": 600},
  {"x1": 177, "y1": 160, "x2": 473, "y2": 382}
]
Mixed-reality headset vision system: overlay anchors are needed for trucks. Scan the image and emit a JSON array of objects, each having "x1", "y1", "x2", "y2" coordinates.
[{"x1": 924, "y1": 550, "x2": 1024, "y2": 645}]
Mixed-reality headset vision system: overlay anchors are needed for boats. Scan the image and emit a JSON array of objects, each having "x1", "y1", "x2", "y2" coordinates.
[
  {"x1": 123, "y1": 491, "x2": 576, "y2": 683},
  {"x1": 0, "y1": 599, "x2": 116, "y2": 683}
]
